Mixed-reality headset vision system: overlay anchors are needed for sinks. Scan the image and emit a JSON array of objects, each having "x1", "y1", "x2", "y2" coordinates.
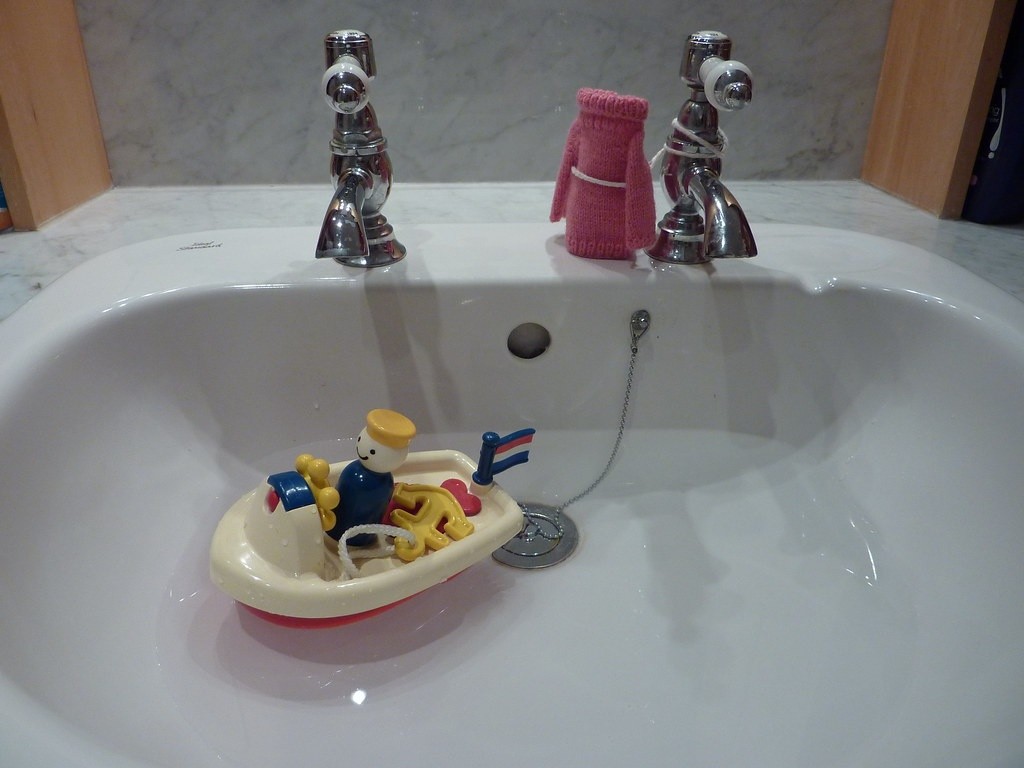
[{"x1": 1, "y1": 219, "x2": 1023, "y2": 766}]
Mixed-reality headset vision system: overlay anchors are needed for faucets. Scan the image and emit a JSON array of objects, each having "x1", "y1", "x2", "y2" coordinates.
[
  {"x1": 643, "y1": 30, "x2": 758, "y2": 265},
  {"x1": 315, "y1": 28, "x2": 407, "y2": 269}
]
